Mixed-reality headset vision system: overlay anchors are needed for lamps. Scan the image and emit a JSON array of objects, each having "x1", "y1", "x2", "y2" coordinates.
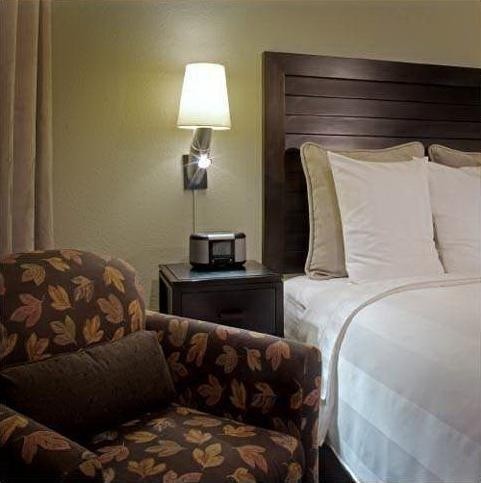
[{"x1": 176, "y1": 60, "x2": 235, "y2": 193}]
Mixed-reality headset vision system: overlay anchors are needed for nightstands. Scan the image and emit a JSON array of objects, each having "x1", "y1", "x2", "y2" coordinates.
[{"x1": 157, "y1": 262, "x2": 288, "y2": 338}]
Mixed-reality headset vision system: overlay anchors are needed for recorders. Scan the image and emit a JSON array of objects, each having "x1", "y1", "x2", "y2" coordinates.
[{"x1": 189, "y1": 232, "x2": 247, "y2": 271}]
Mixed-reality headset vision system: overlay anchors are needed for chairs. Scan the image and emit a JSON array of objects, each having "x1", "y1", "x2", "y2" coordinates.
[{"x1": 0, "y1": 245, "x2": 326, "y2": 483}]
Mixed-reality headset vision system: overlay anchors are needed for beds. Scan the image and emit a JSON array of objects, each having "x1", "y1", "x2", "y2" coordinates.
[{"x1": 258, "y1": 48, "x2": 481, "y2": 483}]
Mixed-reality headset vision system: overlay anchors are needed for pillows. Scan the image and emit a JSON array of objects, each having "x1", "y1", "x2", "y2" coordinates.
[
  {"x1": 2, "y1": 328, "x2": 177, "y2": 437},
  {"x1": 295, "y1": 138, "x2": 481, "y2": 291}
]
